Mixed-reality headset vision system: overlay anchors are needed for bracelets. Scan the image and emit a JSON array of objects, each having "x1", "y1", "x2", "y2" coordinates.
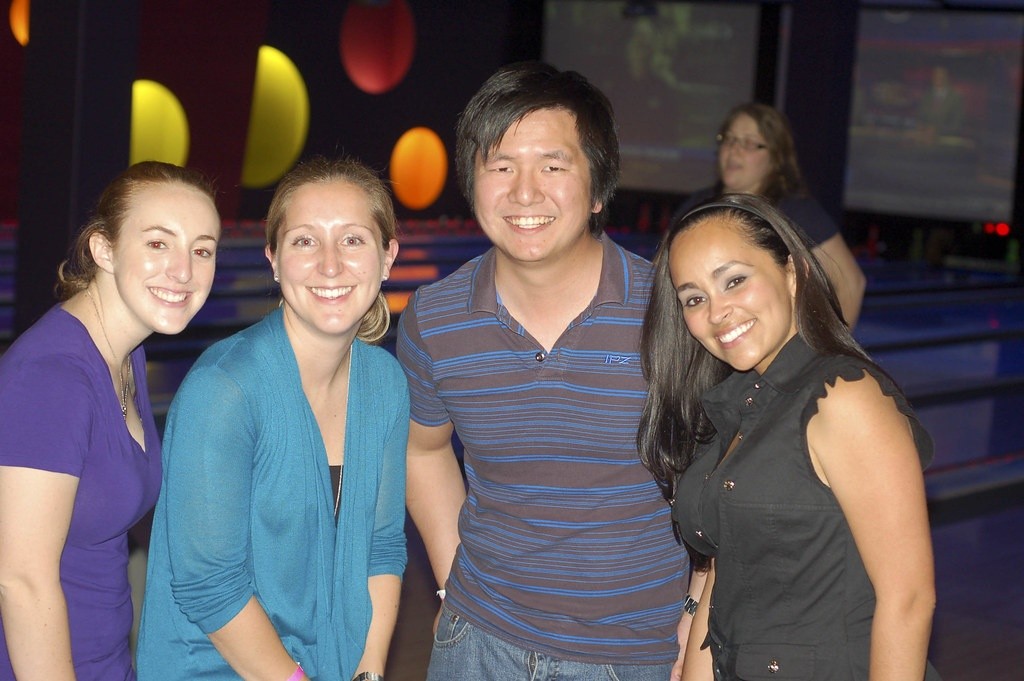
[
  {"x1": 683, "y1": 594, "x2": 698, "y2": 616},
  {"x1": 287, "y1": 664, "x2": 305, "y2": 681},
  {"x1": 436, "y1": 589, "x2": 446, "y2": 603},
  {"x1": 353, "y1": 671, "x2": 385, "y2": 681}
]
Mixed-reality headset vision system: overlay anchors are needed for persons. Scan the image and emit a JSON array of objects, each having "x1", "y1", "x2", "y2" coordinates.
[
  {"x1": 718, "y1": 105, "x2": 865, "y2": 335},
  {"x1": 135, "y1": 154, "x2": 399, "y2": 681},
  {"x1": 0, "y1": 158, "x2": 221, "y2": 681},
  {"x1": 629, "y1": 193, "x2": 941, "y2": 681},
  {"x1": 396, "y1": 63, "x2": 709, "y2": 681}
]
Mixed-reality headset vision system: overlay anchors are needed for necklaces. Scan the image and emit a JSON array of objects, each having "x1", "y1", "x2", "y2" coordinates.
[
  {"x1": 86, "y1": 287, "x2": 131, "y2": 422},
  {"x1": 332, "y1": 343, "x2": 352, "y2": 516}
]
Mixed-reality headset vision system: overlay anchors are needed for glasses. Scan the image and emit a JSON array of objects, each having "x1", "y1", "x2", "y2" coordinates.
[{"x1": 716, "y1": 134, "x2": 769, "y2": 152}]
